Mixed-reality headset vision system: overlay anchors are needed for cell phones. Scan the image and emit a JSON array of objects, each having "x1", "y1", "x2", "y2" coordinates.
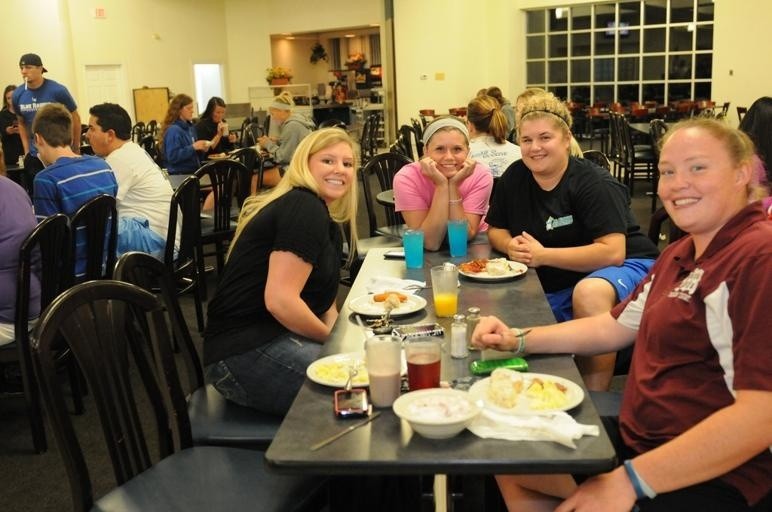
[
  {"x1": 470, "y1": 358, "x2": 529, "y2": 376},
  {"x1": 384, "y1": 250, "x2": 404, "y2": 258},
  {"x1": 392, "y1": 323, "x2": 445, "y2": 340},
  {"x1": 335, "y1": 389, "x2": 369, "y2": 419}
]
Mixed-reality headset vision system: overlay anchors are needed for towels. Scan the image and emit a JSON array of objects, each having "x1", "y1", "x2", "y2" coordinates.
[
  {"x1": 467, "y1": 409, "x2": 603, "y2": 452},
  {"x1": 366, "y1": 271, "x2": 429, "y2": 298}
]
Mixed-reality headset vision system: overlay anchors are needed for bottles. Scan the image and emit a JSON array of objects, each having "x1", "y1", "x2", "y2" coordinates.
[
  {"x1": 449, "y1": 311, "x2": 467, "y2": 363},
  {"x1": 467, "y1": 305, "x2": 484, "y2": 353}
]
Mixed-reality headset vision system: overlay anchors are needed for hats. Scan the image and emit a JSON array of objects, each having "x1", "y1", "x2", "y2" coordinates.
[{"x1": 19, "y1": 53, "x2": 50, "y2": 74}]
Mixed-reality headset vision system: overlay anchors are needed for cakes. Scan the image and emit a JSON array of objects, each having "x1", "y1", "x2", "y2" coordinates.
[{"x1": 488, "y1": 362, "x2": 571, "y2": 417}]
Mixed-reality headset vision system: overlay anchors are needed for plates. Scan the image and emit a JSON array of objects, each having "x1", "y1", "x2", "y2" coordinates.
[
  {"x1": 207, "y1": 155, "x2": 229, "y2": 162},
  {"x1": 304, "y1": 353, "x2": 408, "y2": 389},
  {"x1": 457, "y1": 260, "x2": 528, "y2": 283},
  {"x1": 468, "y1": 371, "x2": 586, "y2": 417},
  {"x1": 348, "y1": 293, "x2": 428, "y2": 320}
]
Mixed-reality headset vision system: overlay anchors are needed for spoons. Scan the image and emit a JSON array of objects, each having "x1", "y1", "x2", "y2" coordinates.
[{"x1": 502, "y1": 329, "x2": 532, "y2": 338}]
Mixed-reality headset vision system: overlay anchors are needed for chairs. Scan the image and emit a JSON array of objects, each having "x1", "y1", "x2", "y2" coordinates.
[
  {"x1": 736, "y1": 107, "x2": 748, "y2": 122},
  {"x1": 655, "y1": 105, "x2": 673, "y2": 118},
  {"x1": 131, "y1": 121, "x2": 142, "y2": 145},
  {"x1": 146, "y1": 121, "x2": 160, "y2": 134},
  {"x1": 449, "y1": 107, "x2": 466, "y2": 116},
  {"x1": 621, "y1": 114, "x2": 661, "y2": 197},
  {"x1": 676, "y1": 100, "x2": 694, "y2": 115},
  {"x1": 630, "y1": 107, "x2": 650, "y2": 115},
  {"x1": 612, "y1": 111, "x2": 655, "y2": 186},
  {"x1": 110, "y1": 251, "x2": 320, "y2": 451},
  {"x1": 72, "y1": 194, "x2": 121, "y2": 283},
  {"x1": 242, "y1": 124, "x2": 266, "y2": 149},
  {"x1": 590, "y1": 102, "x2": 607, "y2": 109},
  {"x1": 606, "y1": 110, "x2": 631, "y2": 182},
  {"x1": 694, "y1": 98, "x2": 716, "y2": 113},
  {"x1": 568, "y1": 101, "x2": 586, "y2": 141},
  {"x1": 134, "y1": 134, "x2": 156, "y2": 159},
  {"x1": 713, "y1": 101, "x2": 730, "y2": 117},
  {"x1": 588, "y1": 112, "x2": 609, "y2": 154},
  {"x1": 388, "y1": 132, "x2": 412, "y2": 167},
  {"x1": 28, "y1": 277, "x2": 332, "y2": 512},
  {"x1": 136, "y1": 172, "x2": 206, "y2": 357},
  {"x1": 644, "y1": 100, "x2": 656, "y2": 108},
  {"x1": 409, "y1": 113, "x2": 432, "y2": 154},
  {"x1": 644, "y1": 116, "x2": 689, "y2": 245},
  {"x1": 609, "y1": 103, "x2": 627, "y2": 112},
  {"x1": 355, "y1": 112, "x2": 382, "y2": 154},
  {"x1": 395, "y1": 125, "x2": 425, "y2": 163},
  {"x1": 361, "y1": 150, "x2": 419, "y2": 239},
  {"x1": 420, "y1": 109, "x2": 439, "y2": 122},
  {"x1": 222, "y1": 146, "x2": 264, "y2": 192},
  {"x1": 185, "y1": 158, "x2": 250, "y2": 273},
  {"x1": 0, "y1": 212, "x2": 87, "y2": 456}
]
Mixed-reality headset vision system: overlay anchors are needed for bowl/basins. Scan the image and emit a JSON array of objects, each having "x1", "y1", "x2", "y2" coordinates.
[{"x1": 392, "y1": 387, "x2": 482, "y2": 441}]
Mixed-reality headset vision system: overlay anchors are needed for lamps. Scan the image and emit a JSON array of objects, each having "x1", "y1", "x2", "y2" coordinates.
[{"x1": 186, "y1": 59, "x2": 235, "y2": 121}]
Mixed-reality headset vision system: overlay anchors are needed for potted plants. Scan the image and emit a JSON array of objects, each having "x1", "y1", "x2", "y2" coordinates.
[{"x1": 263, "y1": 65, "x2": 294, "y2": 88}]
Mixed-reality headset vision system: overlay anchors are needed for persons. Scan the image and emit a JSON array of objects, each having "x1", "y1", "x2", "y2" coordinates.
[
  {"x1": 514, "y1": 89, "x2": 583, "y2": 158},
  {"x1": 464, "y1": 95, "x2": 522, "y2": 178},
  {"x1": 249, "y1": 89, "x2": 312, "y2": 198},
  {"x1": 11, "y1": 53, "x2": 81, "y2": 189},
  {"x1": 202, "y1": 127, "x2": 357, "y2": 420},
  {"x1": 32, "y1": 103, "x2": 116, "y2": 278},
  {"x1": 470, "y1": 118, "x2": 772, "y2": 512},
  {"x1": 84, "y1": 102, "x2": 183, "y2": 265},
  {"x1": 488, "y1": 86, "x2": 515, "y2": 139},
  {"x1": 475, "y1": 87, "x2": 512, "y2": 106},
  {"x1": 486, "y1": 92, "x2": 661, "y2": 391},
  {"x1": 0, "y1": 85, "x2": 25, "y2": 182},
  {"x1": 0, "y1": 142, "x2": 42, "y2": 347},
  {"x1": 158, "y1": 93, "x2": 225, "y2": 218},
  {"x1": 391, "y1": 113, "x2": 494, "y2": 252},
  {"x1": 195, "y1": 96, "x2": 238, "y2": 163}
]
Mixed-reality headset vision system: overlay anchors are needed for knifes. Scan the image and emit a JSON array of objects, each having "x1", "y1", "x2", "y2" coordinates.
[{"x1": 310, "y1": 410, "x2": 382, "y2": 452}]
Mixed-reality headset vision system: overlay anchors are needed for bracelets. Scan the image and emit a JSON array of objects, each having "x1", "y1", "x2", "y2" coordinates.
[
  {"x1": 448, "y1": 196, "x2": 463, "y2": 206},
  {"x1": 511, "y1": 325, "x2": 526, "y2": 355},
  {"x1": 622, "y1": 458, "x2": 661, "y2": 501}
]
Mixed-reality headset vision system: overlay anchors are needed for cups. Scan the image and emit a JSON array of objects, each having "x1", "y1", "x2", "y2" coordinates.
[
  {"x1": 365, "y1": 334, "x2": 403, "y2": 409},
  {"x1": 403, "y1": 336, "x2": 442, "y2": 392},
  {"x1": 221, "y1": 123, "x2": 229, "y2": 137},
  {"x1": 430, "y1": 265, "x2": 458, "y2": 318},
  {"x1": 403, "y1": 227, "x2": 425, "y2": 270},
  {"x1": 447, "y1": 220, "x2": 468, "y2": 258}
]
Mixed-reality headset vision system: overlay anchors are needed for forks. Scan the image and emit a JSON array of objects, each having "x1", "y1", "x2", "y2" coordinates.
[{"x1": 344, "y1": 365, "x2": 357, "y2": 392}]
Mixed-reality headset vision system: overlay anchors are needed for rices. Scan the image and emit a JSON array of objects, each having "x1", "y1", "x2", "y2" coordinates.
[{"x1": 403, "y1": 387, "x2": 477, "y2": 425}]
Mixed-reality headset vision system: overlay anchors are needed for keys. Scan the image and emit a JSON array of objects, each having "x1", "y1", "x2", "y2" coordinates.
[{"x1": 364, "y1": 314, "x2": 400, "y2": 334}]
